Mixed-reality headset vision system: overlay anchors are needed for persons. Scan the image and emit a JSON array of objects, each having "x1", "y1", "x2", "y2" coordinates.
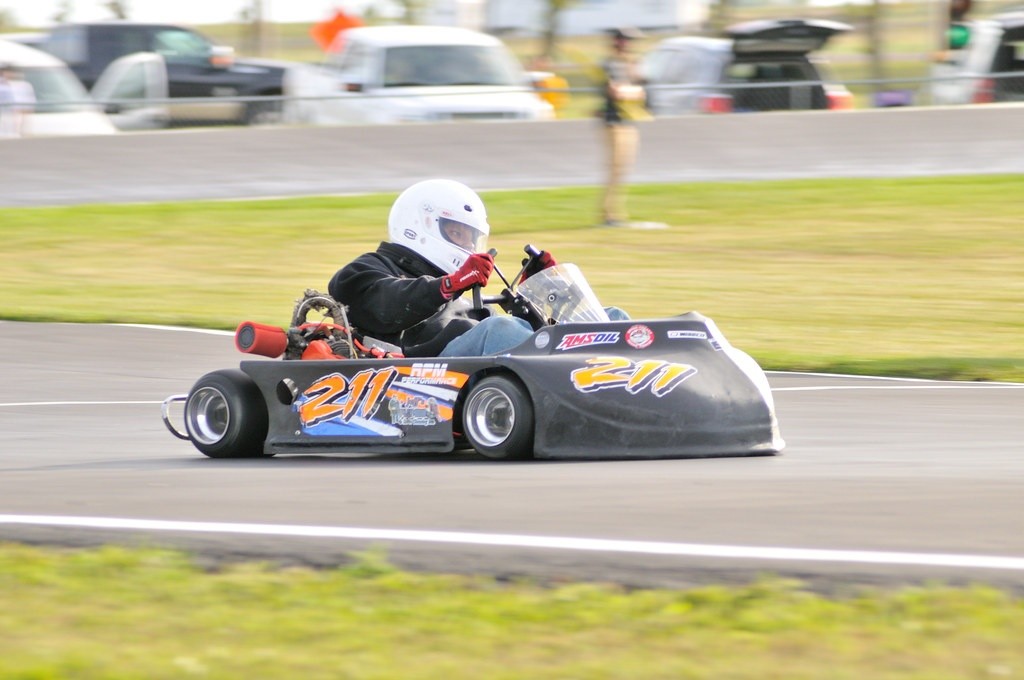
[
  {"x1": 932, "y1": 0, "x2": 975, "y2": 105},
  {"x1": 0, "y1": 62, "x2": 35, "y2": 137},
  {"x1": 603, "y1": 28, "x2": 647, "y2": 226},
  {"x1": 327, "y1": 178, "x2": 630, "y2": 359}
]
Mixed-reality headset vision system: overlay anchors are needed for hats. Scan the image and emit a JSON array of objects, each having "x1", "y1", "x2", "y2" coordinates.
[{"x1": 617, "y1": 26, "x2": 648, "y2": 41}]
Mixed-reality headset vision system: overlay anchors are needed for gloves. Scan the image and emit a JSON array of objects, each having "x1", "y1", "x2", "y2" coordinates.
[
  {"x1": 520, "y1": 252, "x2": 556, "y2": 287},
  {"x1": 440, "y1": 253, "x2": 494, "y2": 299}
]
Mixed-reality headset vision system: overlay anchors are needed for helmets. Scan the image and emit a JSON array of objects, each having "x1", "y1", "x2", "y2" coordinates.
[{"x1": 389, "y1": 180, "x2": 490, "y2": 275}]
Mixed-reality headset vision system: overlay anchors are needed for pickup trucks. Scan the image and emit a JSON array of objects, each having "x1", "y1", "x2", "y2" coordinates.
[
  {"x1": 10, "y1": 26, "x2": 286, "y2": 126},
  {"x1": 278, "y1": 24, "x2": 559, "y2": 128}
]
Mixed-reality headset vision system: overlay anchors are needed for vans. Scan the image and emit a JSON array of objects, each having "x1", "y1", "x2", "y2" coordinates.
[
  {"x1": 0, "y1": 38, "x2": 171, "y2": 137},
  {"x1": 923, "y1": 13, "x2": 1024, "y2": 104},
  {"x1": 623, "y1": 17, "x2": 856, "y2": 117}
]
